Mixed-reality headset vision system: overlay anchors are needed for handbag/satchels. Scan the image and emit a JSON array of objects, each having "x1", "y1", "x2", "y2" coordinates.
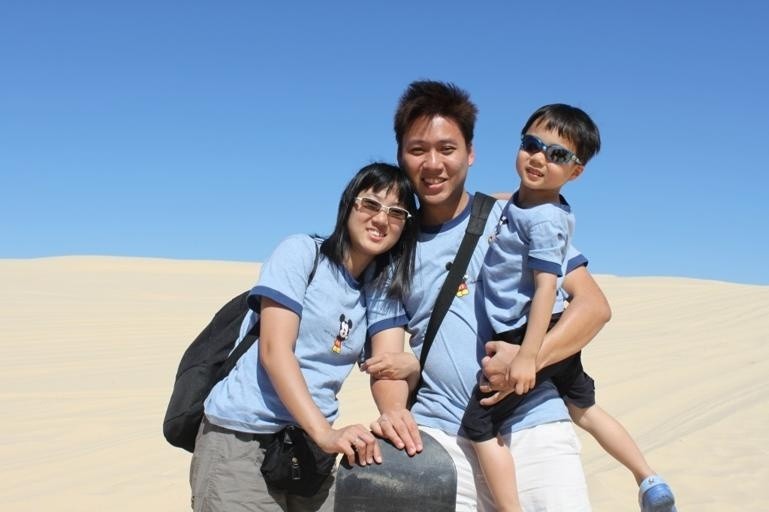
[{"x1": 260, "y1": 425, "x2": 336, "y2": 497}]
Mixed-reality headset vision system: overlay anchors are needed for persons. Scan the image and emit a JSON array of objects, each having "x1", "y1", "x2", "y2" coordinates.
[
  {"x1": 461, "y1": 101, "x2": 678, "y2": 512},
  {"x1": 188, "y1": 161, "x2": 425, "y2": 512},
  {"x1": 365, "y1": 87, "x2": 614, "y2": 512}
]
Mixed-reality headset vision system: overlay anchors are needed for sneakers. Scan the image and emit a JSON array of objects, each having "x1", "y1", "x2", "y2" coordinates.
[{"x1": 639, "y1": 476, "x2": 676, "y2": 511}]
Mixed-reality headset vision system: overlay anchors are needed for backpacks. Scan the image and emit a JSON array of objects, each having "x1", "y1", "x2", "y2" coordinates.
[{"x1": 163, "y1": 241, "x2": 319, "y2": 452}]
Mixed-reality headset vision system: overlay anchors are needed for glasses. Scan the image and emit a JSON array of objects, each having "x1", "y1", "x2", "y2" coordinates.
[
  {"x1": 522, "y1": 135, "x2": 582, "y2": 166},
  {"x1": 355, "y1": 197, "x2": 412, "y2": 219}
]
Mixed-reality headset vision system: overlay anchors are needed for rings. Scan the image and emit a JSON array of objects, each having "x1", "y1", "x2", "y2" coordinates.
[{"x1": 352, "y1": 438, "x2": 360, "y2": 446}]
